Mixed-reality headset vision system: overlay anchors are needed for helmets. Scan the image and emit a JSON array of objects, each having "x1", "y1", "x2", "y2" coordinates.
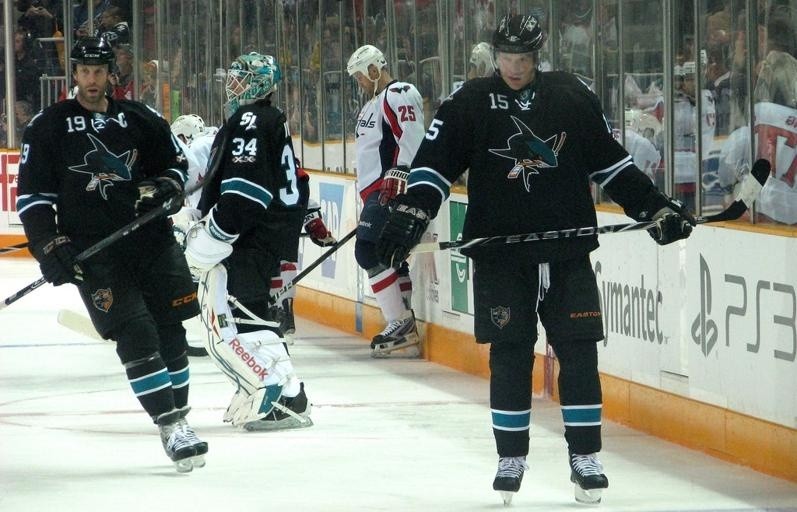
[
  {"x1": 224, "y1": 51, "x2": 282, "y2": 114},
  {"x1": 347, "y1": 44, "x2": 387, "y2": 77},
  {"x1": 69, "y1": 35, "x2": 115, "y2": 66},
  {"x1": 630, "y1": 28, "x2": 731, "y2": 136},
  {"x1": 490, "y1": 14, "x2": 544, "y2": 54},
  {"x1": 470, "y1": 41, "x2": 495, "y2": 79},
  {"x1": 170, "y1": 113, "x2": 206, "y2": 140}
]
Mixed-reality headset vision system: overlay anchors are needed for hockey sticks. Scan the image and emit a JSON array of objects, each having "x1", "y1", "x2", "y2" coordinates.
[
  {"x1": 408, "y1": 160, "x2": 771, "y2": 252},
  {"x1": 1, "y1": 137, "x2": 225, "y2": 310}
]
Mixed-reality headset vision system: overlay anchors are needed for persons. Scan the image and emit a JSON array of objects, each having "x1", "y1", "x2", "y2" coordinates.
[
  {"x1": 342, "y1": 44, "x2": 424, "y2": 363},
  {"x1": 379, "y1": 13, "x2": 693, "y2": 503},
  {"x1": 267, "y1": 162, "x2": 339, "y2": 344},
  {"x1": 169, "y1": 111, "x2": 220, "y2": 212},
  {"x1": 0, "y1": 0, "x2": 135, "y2": 152},
  {"x1": 17, "y1": 37, "x2": 208, "y2": 471},
  {"x1": 187, "y1": 54, "x2": 310, "y2": 429},
  {"x1": 134, "y1": 3, "x2": 797, "y2": 220}
]
